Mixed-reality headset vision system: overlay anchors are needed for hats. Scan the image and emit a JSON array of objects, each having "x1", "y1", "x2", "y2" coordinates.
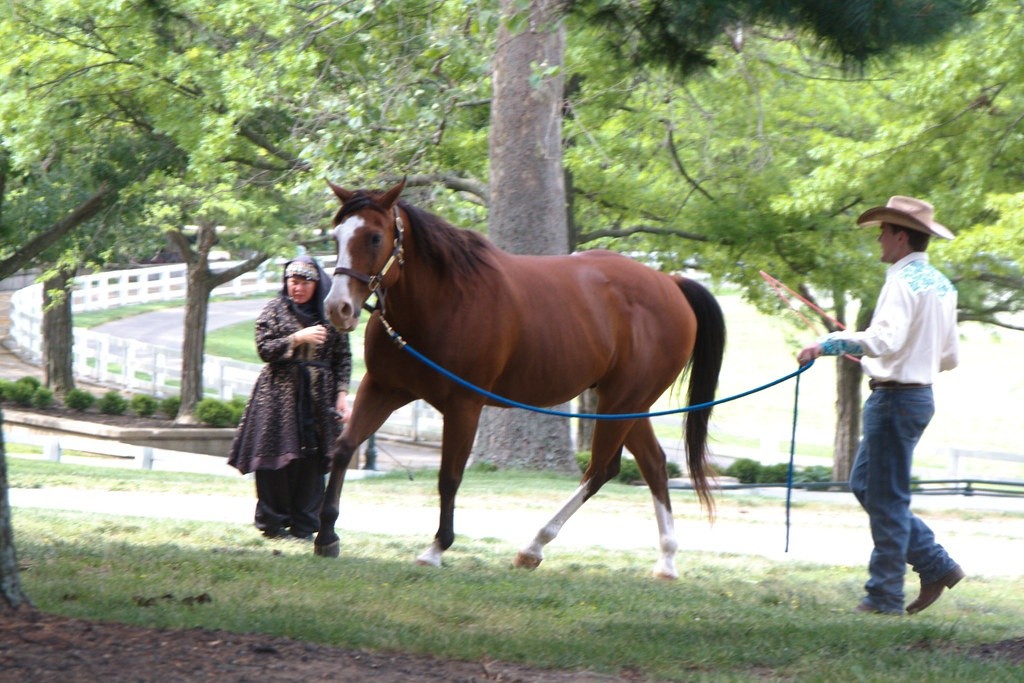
[
  {"x1": 857, "y1": 195, "x2": 956, "y2": 240},
  {"x1": 285, "y1": 261, "x2": 320, "y2": 283}
]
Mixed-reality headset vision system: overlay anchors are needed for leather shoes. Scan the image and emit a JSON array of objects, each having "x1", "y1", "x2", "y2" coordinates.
[
  {"x1": 854, "y1": 601, "x2": 901, "y2": 616},
  {"x1": 907, "y1": 565, "x2": 964, "y2": 614}
]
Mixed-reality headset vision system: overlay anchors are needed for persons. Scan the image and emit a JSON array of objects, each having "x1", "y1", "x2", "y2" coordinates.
[
  {"x1": 226, "y1": 256, "x2": 352, "y2": 539},
  {"x1": 799, "y1": 194, "x2": 965, "y2": 616}
]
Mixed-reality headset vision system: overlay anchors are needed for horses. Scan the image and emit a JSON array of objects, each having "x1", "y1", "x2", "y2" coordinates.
[{"x1": 313, "y1": 173, "x2": 726, "y2": 582}]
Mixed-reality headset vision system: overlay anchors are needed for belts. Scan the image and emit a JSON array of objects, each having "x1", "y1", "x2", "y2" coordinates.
[{"x1": 869, "y1": 380, "x2": 931, "y2": 389}]
